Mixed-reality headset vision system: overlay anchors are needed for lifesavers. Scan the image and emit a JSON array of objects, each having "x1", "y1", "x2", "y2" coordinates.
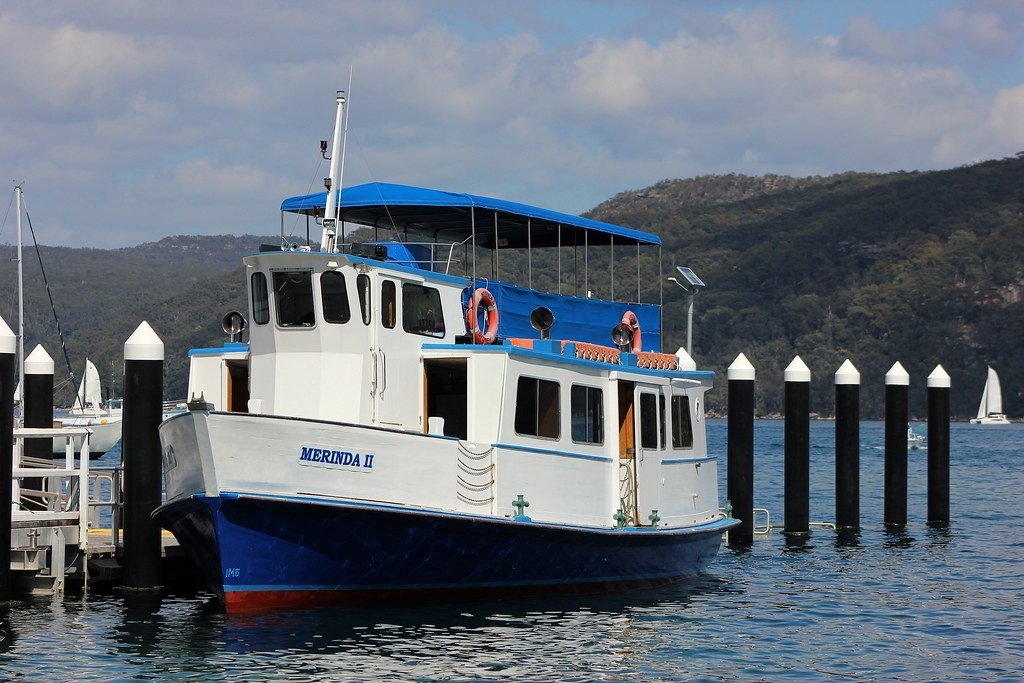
[
  {"x1": 622, "y1": 310, "x2": 641, "y2": 353},
  {"x1": 469, "y1": 288, "x2": 499, "y2": 344}
]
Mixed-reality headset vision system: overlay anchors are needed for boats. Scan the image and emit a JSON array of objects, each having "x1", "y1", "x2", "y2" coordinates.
[
  {"x1": 151, "y1": 63, "x2": 748, "y2": 619},
  {"x1": 908, "y1": 423, "x2": 924, "y2": 451},
  {"x1": 53, "y1": 398, "x2": 189, "y2": 426}
]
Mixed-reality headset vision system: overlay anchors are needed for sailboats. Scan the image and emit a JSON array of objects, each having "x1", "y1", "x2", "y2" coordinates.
[
  {"x1": 1, "y1": 175, "x2": 123, "y2": 462},
  {"x1": 68, "y1": 357, "x2": 109, "y2": 418},
  {"x1": 969, "y1": 365, "x2": 1011, "y2": 426}
]
